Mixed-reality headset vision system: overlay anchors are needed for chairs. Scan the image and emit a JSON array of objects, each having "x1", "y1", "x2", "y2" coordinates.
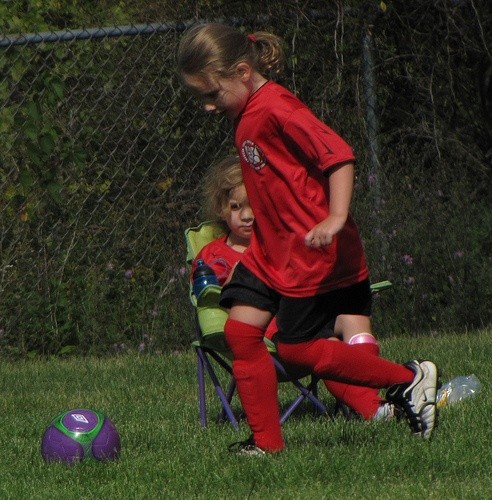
[{"x1": 185, "y1": 220, "x2": 328, "y2": 426}]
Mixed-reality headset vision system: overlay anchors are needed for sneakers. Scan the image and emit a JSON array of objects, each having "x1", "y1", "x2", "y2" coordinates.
[
  {"x1": 372, "y1": 399, "x2": 395, "y2": 433},
  {"x1": 387, "y1": 358, "x2": 439, "y2": 442},
  {"x1": 229, "y1": 435, "x2": 266, "y2": 457}
]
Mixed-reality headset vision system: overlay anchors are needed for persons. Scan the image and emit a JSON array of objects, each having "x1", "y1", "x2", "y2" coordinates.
[
  {"x1": 174, "y1": 22, "x2": 438, "y2": 458},
  {"x1": 190, "y1": 165, "x2": 396, "y2": 426}
]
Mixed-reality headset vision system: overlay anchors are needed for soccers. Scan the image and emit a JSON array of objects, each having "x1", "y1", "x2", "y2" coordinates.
[{"x1": 40, "y1": 407, "x2": 124, "y2": 466}]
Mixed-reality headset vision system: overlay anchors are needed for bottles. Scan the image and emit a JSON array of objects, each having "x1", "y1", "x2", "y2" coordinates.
[{"x1": 193, "y1": 259, "x2": 228, "y2": 300}]
